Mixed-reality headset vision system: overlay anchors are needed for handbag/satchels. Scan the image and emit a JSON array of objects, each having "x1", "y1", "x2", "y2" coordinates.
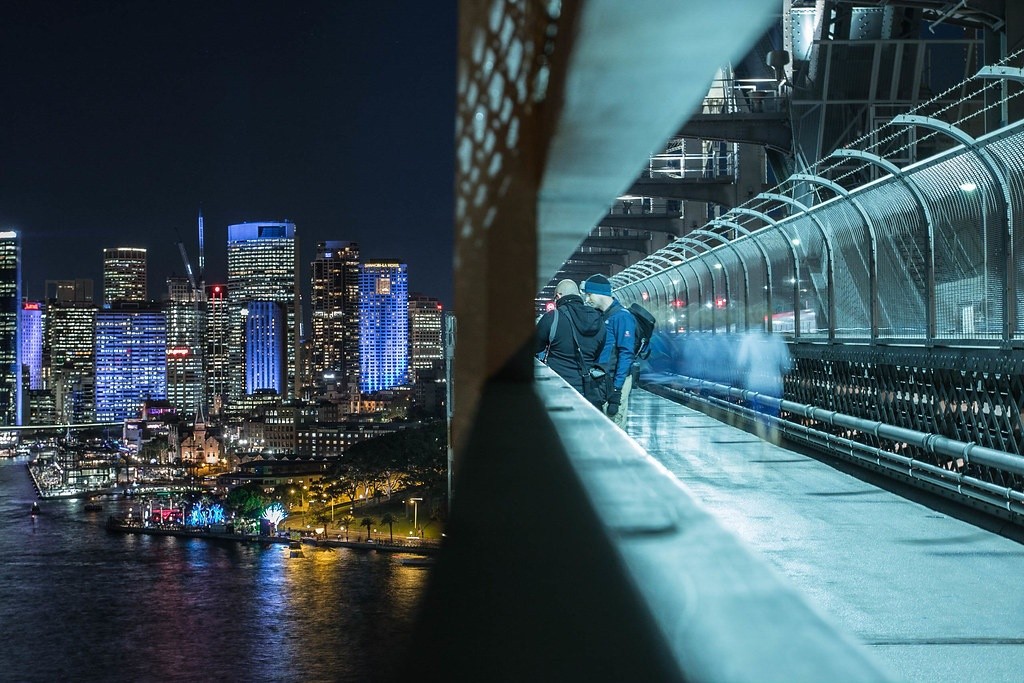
[{"x1": 583, "y1": 363, "x2": 614, "y2": 404}]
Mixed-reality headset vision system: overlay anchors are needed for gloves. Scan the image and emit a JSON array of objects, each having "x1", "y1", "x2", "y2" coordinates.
[{"x1": 607, "y1": 391, "x2": 621, "y2": 414}]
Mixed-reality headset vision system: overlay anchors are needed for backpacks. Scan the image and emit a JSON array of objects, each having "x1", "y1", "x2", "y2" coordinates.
[{"x1": 628, "y1": 303, "x2": 656, "y2": 360}]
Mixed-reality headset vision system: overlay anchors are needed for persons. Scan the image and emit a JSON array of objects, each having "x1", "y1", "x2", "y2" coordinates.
[
  {"x1": 585, "y1": 276, "x2": 636, "y2": 431},
  {"x1": 735, "y1": 302, "x2": 794, "y2": 456},
  {"x1": 534, "y1": 278, "x2": 607, "y2": 414}
]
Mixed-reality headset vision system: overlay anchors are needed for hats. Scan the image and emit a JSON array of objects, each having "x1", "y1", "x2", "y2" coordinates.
[{"x1": 585, "y1": 274, "x2": 612, "y2": 296}]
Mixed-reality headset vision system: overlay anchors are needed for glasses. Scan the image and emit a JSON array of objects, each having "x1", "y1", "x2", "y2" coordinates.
[{"x1": 553, "y1": 293, "x2": 561, "y2": 302}]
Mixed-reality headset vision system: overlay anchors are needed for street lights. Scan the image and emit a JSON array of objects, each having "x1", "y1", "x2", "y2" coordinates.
[{"x1": 401, "y1": 499, "x2": 416, "y2": 535}]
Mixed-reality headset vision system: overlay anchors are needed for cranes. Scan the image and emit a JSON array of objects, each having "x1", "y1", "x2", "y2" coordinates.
[
  {"x1": 197, "y1": 206, "x2": 206, "y2": 284},
  {"x1": 178, "y1": 240, "x2": 198, "y2": 290}
]
